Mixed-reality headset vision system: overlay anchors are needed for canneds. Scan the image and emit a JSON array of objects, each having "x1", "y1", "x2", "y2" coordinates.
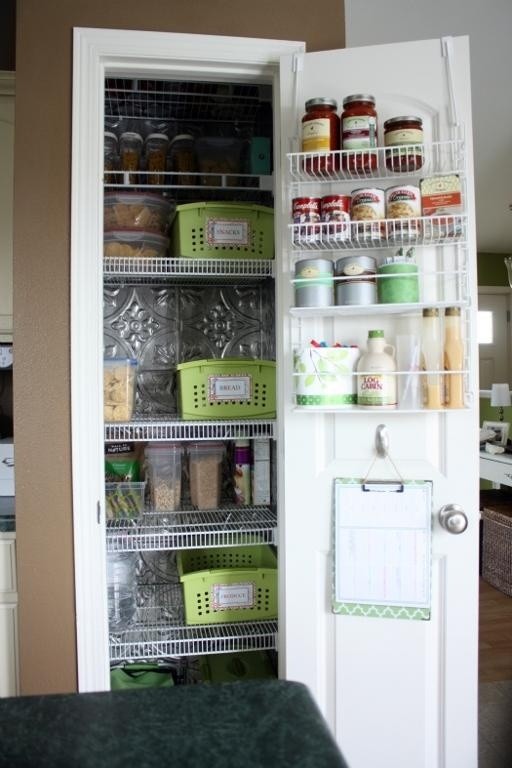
[
  {"x1": 303, "y1": 98, "x2": 340, "y2": 175},
  {"x1": 385, "y1": 115, "x2": 425, "y2": 172},
  {"x1": 341, "y1": 93, "x2": 378, "y2": 174}
]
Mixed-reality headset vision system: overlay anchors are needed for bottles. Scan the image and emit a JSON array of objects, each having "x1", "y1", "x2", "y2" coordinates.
[
  {"x1": 443, "y1": 307, "x2": 463, "y2": 408},
  {"x1": 105, "y1": 132, "x2": 118, "y2": 184},
  {"x1": 120, "y1": 133, "x2": 143, "y2": 184},
  {"x1": 146, "y1": 133, "x2": 167, "y2": 185},
  {"x1": 420, "y1": 307, "x2": 444, "y2": 408},
  {"x1": 175, "y1": 135, "x2": 197, "y2": 189}
]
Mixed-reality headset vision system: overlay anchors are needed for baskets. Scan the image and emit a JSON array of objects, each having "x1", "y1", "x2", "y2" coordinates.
[
  {"x1": 175, "y1": 534, "x2": 277, "y2": 624},
  {"x1": 171, "y1": 202, "x2": 274, "y2": 261},
  {"x1": 173, "y1": 357, "x2": 276, "y2": 420}
]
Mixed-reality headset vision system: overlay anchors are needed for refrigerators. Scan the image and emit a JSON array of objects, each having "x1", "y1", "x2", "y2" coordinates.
[{"x1": 14, "y1": 0, "x2": 477, "y2": 768}]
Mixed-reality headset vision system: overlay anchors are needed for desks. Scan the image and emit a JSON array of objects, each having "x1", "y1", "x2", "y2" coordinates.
[{"x1": 0, "y1": 680, "x2": 349, "y2": 768}]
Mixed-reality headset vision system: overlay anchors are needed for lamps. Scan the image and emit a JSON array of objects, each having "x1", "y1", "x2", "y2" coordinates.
[{"x1": 490, "y1": 382, "x2": 511, "y2": 421}]
